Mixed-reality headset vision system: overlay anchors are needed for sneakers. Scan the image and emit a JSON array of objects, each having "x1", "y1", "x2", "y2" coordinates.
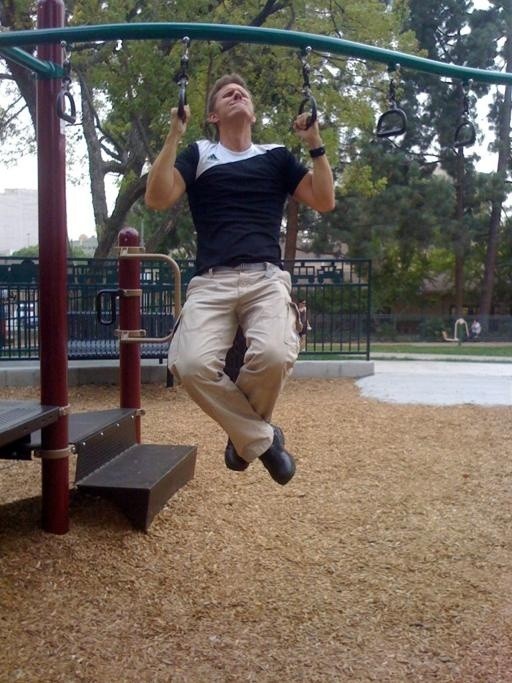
[
  {"x1": 259, "y1": 426, "x2": 295, "y2": 486},
  {"x1": 225, "y1": 437, "x2": 249, "y2": 471}
]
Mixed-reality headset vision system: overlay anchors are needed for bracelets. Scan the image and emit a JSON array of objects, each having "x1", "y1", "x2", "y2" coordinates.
[{"x1": 309, "y1": 145, "x2": 325, "y2": 157}]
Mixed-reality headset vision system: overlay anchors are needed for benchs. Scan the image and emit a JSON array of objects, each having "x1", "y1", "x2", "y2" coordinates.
[{"x1": 66, "y1": 310, "x2": 173, "y2": 364}]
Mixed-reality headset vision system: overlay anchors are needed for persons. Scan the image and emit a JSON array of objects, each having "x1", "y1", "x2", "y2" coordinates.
[
  {"x1": 471, "y1": 319, "x2": 481, "y2": 338},
  {"x1": 297, "y1": 299, "x2": 310, "y2": 352},
  {"x1": 144, "y1": 73, "x2": 335, "y2": 485}
]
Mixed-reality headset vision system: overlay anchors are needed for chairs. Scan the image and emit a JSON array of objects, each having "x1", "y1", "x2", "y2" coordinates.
[{"x1": 443, "y1": 330, "x2": 459, "y2": 342}]
